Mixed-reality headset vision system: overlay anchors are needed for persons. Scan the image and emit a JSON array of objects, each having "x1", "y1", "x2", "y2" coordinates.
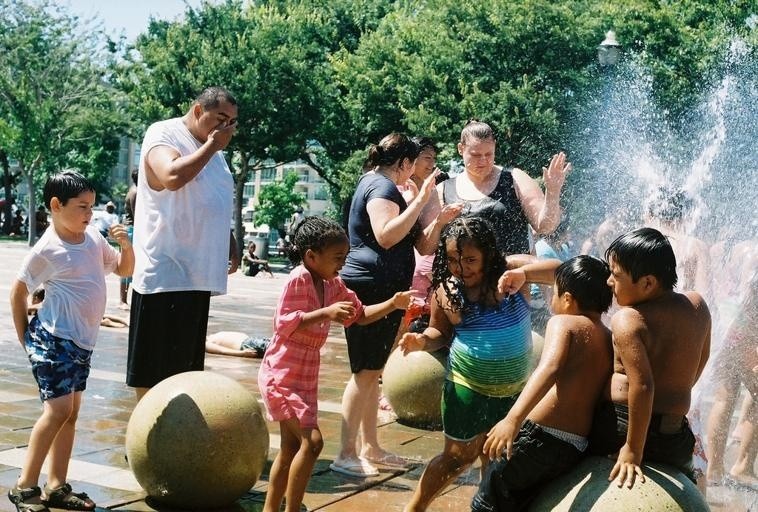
[
  {"x1": 258, "y1": 216, "x2": 420, "y2": 512},
  {"x1": 399, "y1": 217, "x2": 564, "y2": 511},
  {"x1": 204, "y1": 331, "x2": 272, "y2": 358},
  {"x1": 417, "y1": 120, "x2": 570, "y2": 261},
  {"x1": 380, "y1": 138, "x2": 439, "y2": 410},
  {"x1": 289, "y1": 204, "x2": 307, "y2": 243},
  {"x1": 706, "y1": 275, "x2": 758, "y2": 488},
  {"x1": 123, "y1": 86, "x2": 238, "y2": 461},
  {"x1": 574, "y1": 222, "x2": 620, "y2": 264},
  {"x1": 33, "y1": 205, "x2": 49, "y2": 235},
  {"x1": 0, "y1": 196, "x2": 16, "y2": 227},
  {"x1": 643, "y1": 191, "x2": 706, "y2": 298},
  {"x1": 329, "y1": 133, "x2": 441, "y2": 474},
  {"x1": 25, "y1": 289, "x2": 130, "y2": 328},
  {"x1": 241, "y1": 242, "x2": 274, "y2": 277},
  {"x1": 121, "y1": 170, "x2": 138, "y2": 303},
  {"x1": 531, "y1": 211, "x2": 571, "y2": 336},
  {"x1": 470, "y1": 256, "x2": 613, "y2": 511},
  {"x1": 8, "y1": 170, "x2": 135, "y2": 512},
  {"x1": 10, "y1": 208, "x2": 24, "y2": 236},
  {"x1": 592, "y1": 228, "x2": 711, "y2": 487},
  {"x1": 95, "y1": 199, "x2": 120, "y2": 238}
]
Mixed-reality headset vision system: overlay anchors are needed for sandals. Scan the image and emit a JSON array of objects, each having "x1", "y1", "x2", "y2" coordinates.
[
  {"x1": 8, "y1": 487, "x2": 50, "y2": 512},
  {"x1": 41, "y1": 484, "x2": 96, "y2": 510}
]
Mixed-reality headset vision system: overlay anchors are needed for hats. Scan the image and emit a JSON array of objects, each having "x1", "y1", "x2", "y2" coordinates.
[{"x1": 105, "y1": 201, "x2": 116, "y2": 210}]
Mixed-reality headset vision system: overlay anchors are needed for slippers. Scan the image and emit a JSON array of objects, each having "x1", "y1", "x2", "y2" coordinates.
[
  {"x1": 369, "y1": 455, "x2": 408, "y2": 466},
  {"x1": 329, "y1": 464, "x2": 379, "y2": 477}
]
